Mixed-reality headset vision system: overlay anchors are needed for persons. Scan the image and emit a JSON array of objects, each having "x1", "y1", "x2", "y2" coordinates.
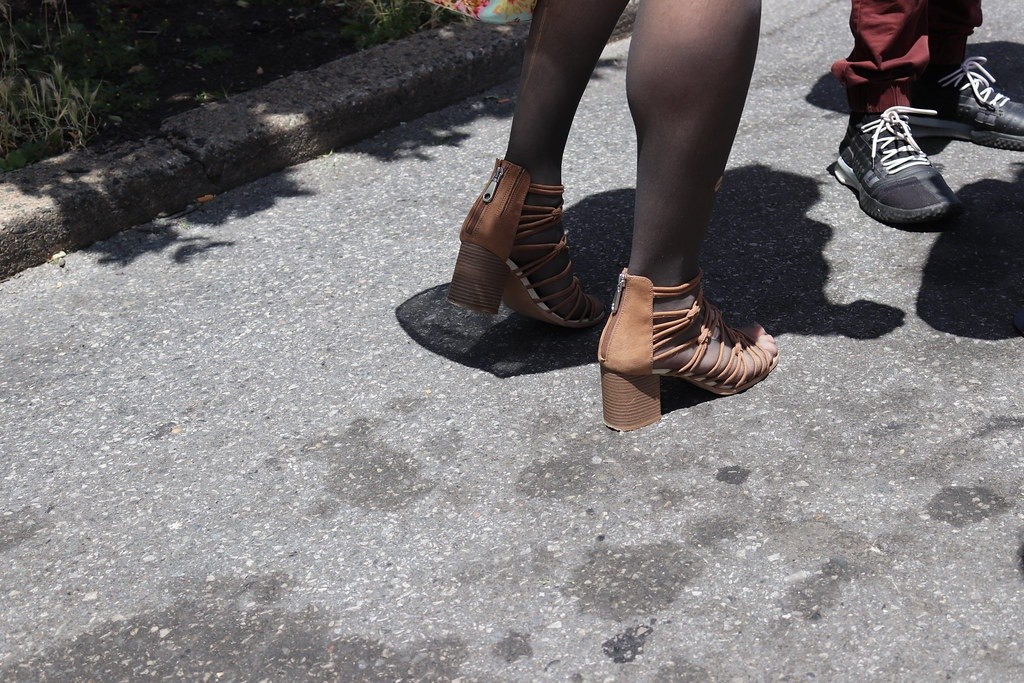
[
  {"x1": 831, "y1": 0, "x2": 1023, "y2": 223},
  {"x1": 446, "y1": 0, "x2": 778, "y2": 431}
]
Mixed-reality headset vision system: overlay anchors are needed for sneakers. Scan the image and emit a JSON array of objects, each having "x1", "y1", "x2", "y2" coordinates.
[
  {"x1": 905, "y1": 56, "x2": 1024, "y2": 151},
  {"x1": 834, "y1": 106, "x2": 960, "y2": 223}
]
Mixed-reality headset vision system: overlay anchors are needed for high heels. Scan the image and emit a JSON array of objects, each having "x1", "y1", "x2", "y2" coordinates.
[
  {"x1": 598, "y1": 266, "x2": 779, "y2": 432},
  {"x1": 445, "y1": 158, "x2": 607, "y2": 328}
]
